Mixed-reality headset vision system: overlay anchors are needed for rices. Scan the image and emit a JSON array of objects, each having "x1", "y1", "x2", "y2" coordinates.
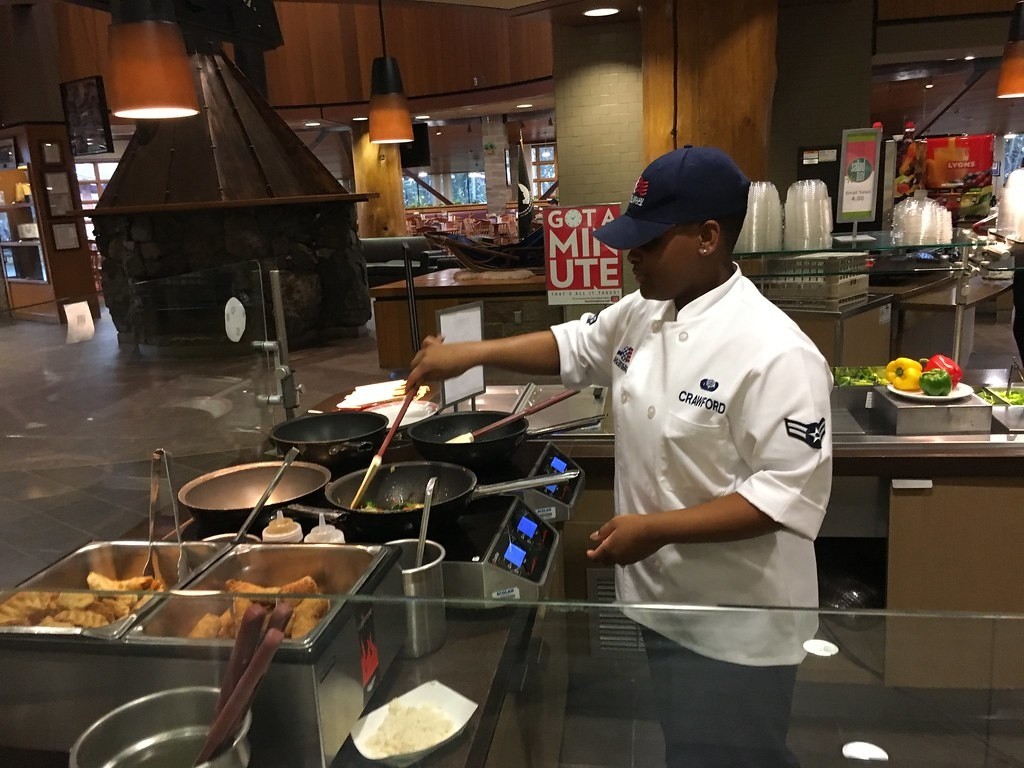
[{"x1": 362, "y1": 699, "x2": 453, "y2": 756}]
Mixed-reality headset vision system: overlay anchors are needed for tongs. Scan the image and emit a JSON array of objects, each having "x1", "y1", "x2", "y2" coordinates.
[{"x1": 142, "y1": 448, "x2": 190, "y2": 584}]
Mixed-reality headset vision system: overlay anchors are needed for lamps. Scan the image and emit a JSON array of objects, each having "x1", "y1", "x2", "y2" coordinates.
[
  {"x1": 108, "y1": 1, "x2": 200, "y2": 119},
  {"x1": 59, "y1": 75, "x2": 114, "y2": 156},
  {"x1": 996, "y1": 0, "x2": 1024, "y2": 98},
  {"x1": 367, "y1": 0, "x2": 414, "y2": 143}
]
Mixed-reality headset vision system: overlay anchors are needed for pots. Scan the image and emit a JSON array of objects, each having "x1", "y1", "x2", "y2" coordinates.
[
  {"x1": 176, "y1": 460, "x2": 331, "y2": 529},
  {"x1": 406, "y1": 410, "x2": 603, "y2": 466},
  {"x1": 324, "y1": 460, "x2": 581, "y2": 527},
  {"x1": 69, "y1": 685, "x2": 253, "y2": 768},
  {"x1": 268, "y1": 409, "x2": 389, "y2": 473}
]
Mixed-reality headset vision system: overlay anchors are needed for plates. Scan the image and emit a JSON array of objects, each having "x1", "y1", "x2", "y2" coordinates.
[
  {"x1": 886, "y1": 381, "x2": 973, "y2": 402},
  {"x1": 362, "y1": 400, "x2": 440, "y2": 432}
]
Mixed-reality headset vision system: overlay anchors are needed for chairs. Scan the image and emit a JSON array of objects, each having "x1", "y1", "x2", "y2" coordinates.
[{"x1": 406, "y1": 212, "x2": 519, "y2": 243}]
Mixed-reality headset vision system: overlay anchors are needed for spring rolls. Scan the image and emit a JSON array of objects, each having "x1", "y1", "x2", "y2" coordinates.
[{"x1": 184, "y1": 576, "x2": 330, "y2": 641}]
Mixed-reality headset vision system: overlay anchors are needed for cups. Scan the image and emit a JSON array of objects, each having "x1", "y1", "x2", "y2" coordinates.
[{"x1": 384, "y1": 537, "x2": 447, "y2": 660}]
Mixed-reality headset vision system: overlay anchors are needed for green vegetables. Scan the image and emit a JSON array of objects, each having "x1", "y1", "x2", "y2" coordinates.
[
  {"x1": 363, "y1": 500, "x2": 416, "y2": 511},
  {"x1": 978, "y1": 388, "x2": 1024, "y2": 407},
  {"x1": 835, "y1": 368, "x2": 890, "y2": 386}
]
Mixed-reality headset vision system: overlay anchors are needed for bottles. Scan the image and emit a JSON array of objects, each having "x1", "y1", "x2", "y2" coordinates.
[
  {"x1": 261, "y1": 510, "x2": 304, "y2": 544},
  {"x1": 303, "y1": 513, "x2": 347, "y2": 545}
]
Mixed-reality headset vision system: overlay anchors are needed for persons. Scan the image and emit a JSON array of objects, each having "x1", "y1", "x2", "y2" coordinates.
[
  {"x1": 405, "y1": 146, "x2": 834, "y2": 768},
  {"x1": 997, "y1": 157, "x2": 1024, "y2": 368}
]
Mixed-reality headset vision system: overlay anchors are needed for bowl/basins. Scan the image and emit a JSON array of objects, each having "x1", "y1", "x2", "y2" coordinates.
[
  {"x1": 349, "y1": 680, "x2": 479, "y2": 768},
  {"x1": 201, "y1": 532, "x2": 261, "y2": 545},
  {"x1": 732, "y1": 178, "x2": 833, "y2": 253}
]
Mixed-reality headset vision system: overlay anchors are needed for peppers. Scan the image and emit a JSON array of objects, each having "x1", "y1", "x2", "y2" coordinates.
[{"x1": 884, "y1": 354, "x2": 963, "y2": 396}]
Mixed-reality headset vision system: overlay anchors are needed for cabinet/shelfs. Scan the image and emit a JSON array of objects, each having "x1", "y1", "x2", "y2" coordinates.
[{"x1": 0, "y1": 162, "x2": 48, "y2": 285}]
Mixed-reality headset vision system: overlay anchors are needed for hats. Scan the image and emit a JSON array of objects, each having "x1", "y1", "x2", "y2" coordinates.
[{"x1": 593, "y1": 144, "x2": 751, "y2": 249}]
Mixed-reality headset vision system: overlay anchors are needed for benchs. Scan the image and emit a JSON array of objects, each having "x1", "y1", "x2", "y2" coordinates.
[{"x1": 359, "y1": 235, "x2": 459, "y2": 286}]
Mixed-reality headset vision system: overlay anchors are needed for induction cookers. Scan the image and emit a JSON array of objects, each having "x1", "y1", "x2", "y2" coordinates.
[
  {"x1": 409, "y1": 493, "x2": 561, "y2": 608},
  {"x1": 471, "y1": 439, "x2": 586, "y2": 523}
]
